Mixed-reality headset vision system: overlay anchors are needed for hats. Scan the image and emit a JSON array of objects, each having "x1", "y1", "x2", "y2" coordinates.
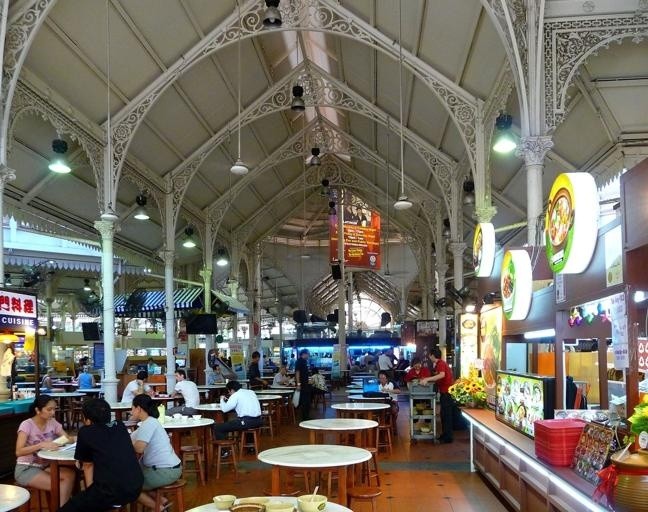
[
  {"x1": 133, "y1": 393, "x2": 160, "y2": 418},
  {"x1": 301, "y1": 349, "x2": 309, "y2": 354}
]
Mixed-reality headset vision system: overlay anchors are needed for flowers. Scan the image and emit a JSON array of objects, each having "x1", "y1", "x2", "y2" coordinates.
[{"x1": 448, "y1": 376, "x2": 488, "y2": 404}]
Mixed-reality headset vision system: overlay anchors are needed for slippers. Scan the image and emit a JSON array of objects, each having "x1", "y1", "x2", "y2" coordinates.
[{"x1": 163, "y1": 502, "x2": 171, "y2": 509}]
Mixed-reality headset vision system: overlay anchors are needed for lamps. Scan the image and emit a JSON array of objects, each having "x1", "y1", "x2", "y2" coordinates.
[
  {"x1": 430, "y1": 242, "x2": 436, "y2": 257},
  {"x1": 291, "y1": 31, "x2": 305, "y2": 112},
  {"x1": 321, "y1": 153, "x2": 330, "y2": 197},
  {"x1": 328, "y1": 198, "x2": 336, "y2": 215},
  {"x1": 273, "y1": 245, "x2": 281, "y2": 305},
  {"x1": 133, "y1": 189, "x2": 150, "y2": 220},
  {"x1": 393, "y1": 0, "x2": 414, "y2": 211},
  {"x1": 492, "y1": 114, "x2": 517, "y2": 156},
  {"x1": 463, "y1": 181, "x2": 475, "y2": 207},
  {"x1": 310, "y1": 106, "x2": 321, "y2": 167},
  {"x1": 183, "y1": 223, "x2": 196, "y2": 248},
  {"x1": 48, "y1": 134, "x2": 72, "y2": 174},
  {"x1": 384, "y1": 116, "x2": 391, "y2": 277},
  {"x1": 84, "y1": 279, "x2": 91, "y2": 291},
  {"x1": 441, "y1": 218, "x2": 451, "y2": 239},
  {"x1": 301, "y1": 115, "x2": 311, "y2": 257},
  {"x1": 217, "y1": 246, "x2": 228, "y2": 267},
  {"x1": 230, "y1": 1, "x2": 250, "y2": 175}
]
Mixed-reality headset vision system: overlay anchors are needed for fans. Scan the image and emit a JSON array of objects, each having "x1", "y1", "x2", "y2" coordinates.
[
  {"x1": 445, "y1": 285, "x2": 470, "y2": 307},
  {"x1": 76, "y1": 288, "x2": 102, "y2": 318},
  {"x1": 124, "y1": 287, "x2": 147, "y2": 321},
  {"x1": 180, "y1": 310, "x2": 194, "y2": 327},
  {"x1": 429, "y1": 296, "x2": 450, "y2": 316},
  {"x1": 211, "y1": 300, "x2": 230, "y2": 318},
  {"x1": 155, "y1": 308, "x2": 166, "y2": 323},
  {"x1": 23, "y1": 258, "x2": 59, "y2": 290}
]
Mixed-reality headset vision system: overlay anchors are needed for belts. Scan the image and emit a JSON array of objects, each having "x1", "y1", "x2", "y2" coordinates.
[{"x1": 173, "y1": 463, "x2": 180, "y2": 469}]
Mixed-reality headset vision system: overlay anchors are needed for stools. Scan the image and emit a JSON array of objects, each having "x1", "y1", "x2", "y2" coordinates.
[
  {"x1": 310, "y1": 469, "x2": 338, "y2": 497},
  {"x1": 278, "y1": 404, "x2": 296, "y2": 424},
  {"x1": 71, "y1": 402, "x2": 84, "y2": 421},
  {"x1": 64, "y1": 405, "x2": 70, "y2": 409},
  {"x1": 385, "y1": 411, "x2": 394, "y2": 431},
  {"x1": 155, "y1": 480, "x2": 187, "y2": 512},
  {"x1": 330, "y1": 377, "x2": 347, "y2": 391},
  {"x1": 346, "y1": 487, "x2": 382, "y2": 512},
  {"x1": 259, "y1": 410, "x2": 276, "y2": 440},
  {"x1": 314, "y1": 393, "x2": 326, "y2": 412},
  {"x1": 285, "y1": 469, "x2": 310, "y2": 493},
  {"x1": 72, "y1": 407, "x2": 83, "y2": 430},
  {"x1": 72, "y1": 401, "x2": 77, "y2": 409},
  {"x1": 109, "y1": 504, "x2": 127, "y2": 512},
  {"x1": 262, "y1": 485, "x2": 301, "y2": 496},
  {"x1": 181, "y1": 445, "x2": 205, "y2": 480},
  {"x1": 321, "y1": 384, "x2": 332, "y2": 399},
  {"x1": 375, "y1": 423, "x2": 392, "y2": 456},
  {"x1": 240, "y1": 428, "x2": 259, "y2": 459},
  {"x1": 14, "y1": 482, "x2": 52, "y2": 512},
  {"x1": 210, "y1": 440, "x2": 238, "y2": 479},
  {"x1": 55, "y1": 409, "x2": 70, "y2": 432},
  {"x1": 79, "y1": 470, "x2": 87, "y2": 491}
]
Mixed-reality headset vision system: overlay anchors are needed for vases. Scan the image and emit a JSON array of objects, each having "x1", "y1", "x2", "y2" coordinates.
[{"x1": 465, "y1": 402, "x2": 478, "y2": 408}]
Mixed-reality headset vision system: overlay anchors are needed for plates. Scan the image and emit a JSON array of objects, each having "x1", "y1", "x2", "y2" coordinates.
[{"x1": 536, "y1": 420, "x2": 586, "y2": 468}]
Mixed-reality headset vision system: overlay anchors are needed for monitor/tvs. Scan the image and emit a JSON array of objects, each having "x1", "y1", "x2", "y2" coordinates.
[
  {"x1": 186, "y1": 314, "x2": 219, "y2": 335},
  {"x1": 82, "y1": 323, "x2": 100, "y2": 342}
]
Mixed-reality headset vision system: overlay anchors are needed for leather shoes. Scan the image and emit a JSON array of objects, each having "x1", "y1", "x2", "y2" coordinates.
[
  {"x1": 247, "y1": 448, "x2": 255, "y2": 455},
  {"x1": 221, "y1": 449, "x2": 230, "y2": 458}
]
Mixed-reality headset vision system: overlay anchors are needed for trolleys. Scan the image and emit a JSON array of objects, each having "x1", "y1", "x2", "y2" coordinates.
[{"x1": 408, "y1": 392, "x2": 440, "y2": 447}]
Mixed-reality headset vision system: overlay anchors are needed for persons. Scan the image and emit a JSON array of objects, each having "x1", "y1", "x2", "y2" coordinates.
[
  {"x1": 376, "y1": 370, "x2": 401, "y2": 436},
  {"x1": 56, "y1": 399, "x2": 144, "y2": 512},
  {"x1": 366, "y1": 351, "x2": 374, "y2": 364},
  {"x1": 147, "y1": 357, "x2": 158, "y2": 374},
  {"x1": 246, "y1": 351, "x2": 268, "y2": 390},
  {"x1": 208, "y1": 365, "x2": 227, "y2": 396},
  {"x1": 404, "y1": 358, "x2": 432, "y2": 430},
  {"x1": 420, "y1": 348, "x2": 456, "y2": 444},
  {"x1": 272, "y1": 366, "x2": 294, "y2": 384},
  {"x1": 289, "y1": 353, "x2": 296, "y2": 370},
  {"x1": 378, "y1": 350, "x2": 393, "y2": 379},
  {"x1": 121, "y1": 371, "x2": 148, "y2": 405},
  {"x1": 129, "y1": 394, "x2": 183, "y2": 512},
  {"x1": 77, "y1": 366, "x2": 97, "y2": 399},
  {"x1": 215, "y1": 380, "x2": 263, "y2": 459},
  {"x1": 14, "y1": 395, "x2": 76, "y2": 508},
  {"x1": 166, "y1": 369, "x2": 200, "y2": 436},
  {"x1": 295, "y1": 349, "x2": 313, "y2": 420},
  {"x1": 387, "y1": 348, "x2": 398, "y2": 365},
  {"x1": 40, "y1": 368, "x2": 55, "y2": 389},
  {"x1": 360, "y1": 352, "x2": 366, "y2": 368},
  {"x1": 78, "y1": 358, "x2": 87, "y2": 368}
]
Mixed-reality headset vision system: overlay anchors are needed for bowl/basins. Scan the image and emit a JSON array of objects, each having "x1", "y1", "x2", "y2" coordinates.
[
  {"x1": 298, "y1": 493, "x2": 328, "y2": 511},
  {"x1": 192, "y1": 415, "x2": 202, "y2": 419},
  {"x1": 211, "y1": 403, "x2": 216, "y2": 408},
  {"x1": 181, "y1": 415, "x2": 188, "y2": 422},
  {"x1": 173, "y1": 413, "x2": 182, "y2": 419},
  {"x1": 240, "y1": 497, "x2": 268, "y2": 504},
  {"x1": 268, "y1": 502, "x2": 295, "y2": 511},
  {"x1": 212, "y1": 494, "x2": 237, "y2": 509},
  {"x1": 229, "y1": 503, "x2": 266, "y2": 512}
]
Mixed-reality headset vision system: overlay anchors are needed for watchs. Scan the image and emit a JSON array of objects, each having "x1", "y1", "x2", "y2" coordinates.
[{"x1": 297, "y1": 383, "x2": 302, "y2": 386}]
